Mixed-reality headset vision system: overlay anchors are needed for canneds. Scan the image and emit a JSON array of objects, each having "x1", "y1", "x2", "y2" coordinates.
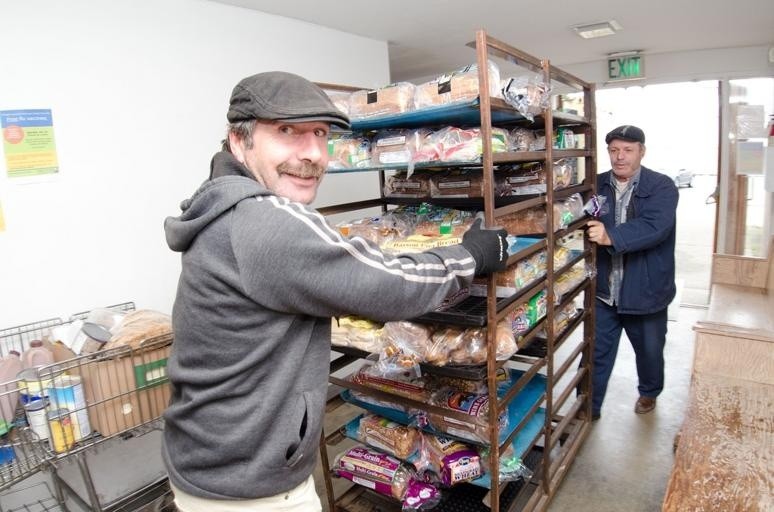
[
  {"x1": 70, "y1": 322, "x2": 112, "y2": 356},
  {"x1": 15, "y1": 364, "x2": 91, "y2": 454}
]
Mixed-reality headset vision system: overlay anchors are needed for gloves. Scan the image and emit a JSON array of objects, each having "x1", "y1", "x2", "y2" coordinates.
[{"x1": 460, "y1": 216, "x2": 509, "y2": 278}]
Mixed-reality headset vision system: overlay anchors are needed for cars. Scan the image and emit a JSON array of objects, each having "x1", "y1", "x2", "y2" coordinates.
[{"x1": 674, "y1": 167, "x2": 695, "y2": 188}]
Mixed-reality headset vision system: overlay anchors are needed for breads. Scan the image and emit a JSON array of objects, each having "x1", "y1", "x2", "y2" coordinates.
[{"x1": 327, "y1": 71, "x2": 584, "y2": 501}]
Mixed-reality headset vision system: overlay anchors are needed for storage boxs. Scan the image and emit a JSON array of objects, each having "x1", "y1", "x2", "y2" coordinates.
[{"x1": 40, "y1": 333, "x2": 174, "y2": 439}]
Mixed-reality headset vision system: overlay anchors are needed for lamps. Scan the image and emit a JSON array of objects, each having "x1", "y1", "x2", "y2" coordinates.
[{"x1": 568, "y1": 19, "x2": 622, "y2": 40}]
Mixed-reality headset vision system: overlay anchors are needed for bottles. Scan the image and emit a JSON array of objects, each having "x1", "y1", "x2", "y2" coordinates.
[{"x1": 0, "y1": 349, "x2": 24, "y2": 421}]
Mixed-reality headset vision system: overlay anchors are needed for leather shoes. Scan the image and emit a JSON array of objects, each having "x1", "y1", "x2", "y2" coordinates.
[{"x1": 635, "y1": 394, "x2": 657, "y2": 415}]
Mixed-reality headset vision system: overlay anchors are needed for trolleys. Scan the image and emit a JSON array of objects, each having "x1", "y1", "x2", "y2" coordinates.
[{"x1": 0, "y1": 301, "x2": 175, "y2": 512}]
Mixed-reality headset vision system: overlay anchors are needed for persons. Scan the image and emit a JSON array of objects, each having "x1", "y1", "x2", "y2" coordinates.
[
  {"x1": 578, "y1": 126, "x2": 677, "y2": 423},
  {"x1": 160, "y1": 71, "x2": 507, "y2": 512}
]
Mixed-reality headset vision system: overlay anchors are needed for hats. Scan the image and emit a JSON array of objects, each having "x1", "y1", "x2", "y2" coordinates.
[
  {"x1": 605, "y1": 124, "x2": 646, "y2": 144},
  {"x1": 228, "y1": 70, "x2": 353, "y2": 132}
]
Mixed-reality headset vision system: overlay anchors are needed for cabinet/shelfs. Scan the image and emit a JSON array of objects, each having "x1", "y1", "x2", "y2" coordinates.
[{"x1": 308, "y1": 28, "x2": 597, "y2": 512}]
